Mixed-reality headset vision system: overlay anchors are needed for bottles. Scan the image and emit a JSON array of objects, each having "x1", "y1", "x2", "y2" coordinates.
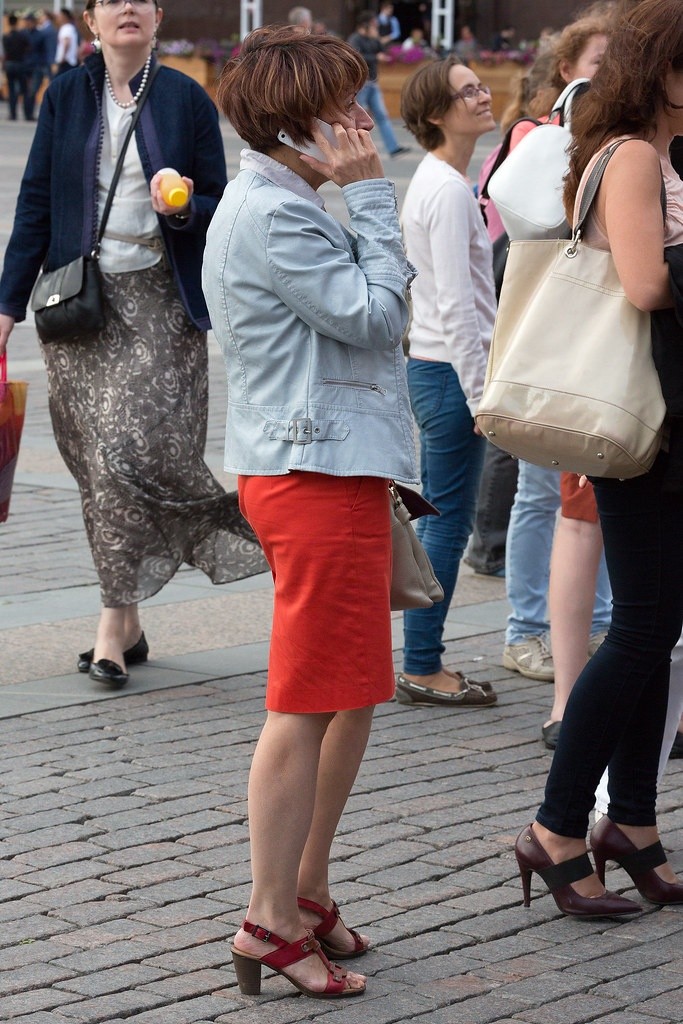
[{"x1": 156, "y1": 168, "x2": 189, "y2": 208}]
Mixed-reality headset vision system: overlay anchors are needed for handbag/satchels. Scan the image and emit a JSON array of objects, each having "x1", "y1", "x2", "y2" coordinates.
[
  {"x1": 475, "y1": 136, "x2": 672, "y2": 478},
  {"x1": 0, "y1": 349, "x2": 28, "y2": 521},
  {"x1": 386, "y1": 480, "x2": 445, "y2": 611},
  {"x1": 31, "y1": 249, "x2": 105, "y2": 343},
  {"x1": 487, "y1": 78, "x2": 590, "y2": 239}
]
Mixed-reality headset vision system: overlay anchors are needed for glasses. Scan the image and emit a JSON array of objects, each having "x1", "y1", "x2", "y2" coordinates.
[
  {"x1": 90, "y1": 0, "x2": 155, "y2": 12},
  {"x1": 449, "y1": 86, "x2": 490, "y2": 100}
]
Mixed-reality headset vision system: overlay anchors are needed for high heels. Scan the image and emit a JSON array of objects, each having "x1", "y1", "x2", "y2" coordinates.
[
  {"x1": 589, "y1": 816, "x2": 683, "y2": 904},
  {"x1": 297, "y1": 896, "x2": 370, "y2": 958},
  {"x1": 516, "y1": 823, "x2": 642, "y2": 917},
  {"x1": 230, "y1": 920, "x2": 367, "y2": 998}
]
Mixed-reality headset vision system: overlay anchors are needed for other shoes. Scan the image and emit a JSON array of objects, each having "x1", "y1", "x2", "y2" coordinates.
[
  {"x1": 542, "y1": 722, "x2": 562, "y2": 749},
  {"x1": 396, "y1": 676, "x2": 497, "y2": 707},
  {"x1": 455, "y1": 671, "x2": 493, "y2": 691},
  {"x1": 668, "y1": 730, "x2": 683, "y2": 759}
]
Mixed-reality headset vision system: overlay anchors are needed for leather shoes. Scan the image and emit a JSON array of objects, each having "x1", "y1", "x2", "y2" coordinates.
[
  {"x1": 90, "y1": 651, "x2": 128, "y2": 686},
  {"x1": 77, "y1": 631, "x2": 148, "y2": 672}
]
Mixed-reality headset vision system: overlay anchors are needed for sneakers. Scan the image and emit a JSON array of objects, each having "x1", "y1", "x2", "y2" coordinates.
[
  {"x1": 587, "y1": 632, "x2": 606, "y2": 659},
  {"x1": 504, "y1": 635, "x2": 554, "y2": 680}
]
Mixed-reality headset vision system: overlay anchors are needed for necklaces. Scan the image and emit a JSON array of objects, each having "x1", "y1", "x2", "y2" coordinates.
[{"x1": 106, "y1": 55, "x2": 151, "y2": 108}]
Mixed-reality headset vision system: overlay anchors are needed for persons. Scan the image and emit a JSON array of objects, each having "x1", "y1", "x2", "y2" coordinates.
[
  {"x1": 346, "y1": 11, "x2": 411, "y2": 161},
  {"x1": 0, "y1": 0, "x2": 270, "y2": 687},
  {"x1": 492, "y1": 24, "x2": 515, "y2": 53},
  {"x1": 462, "y1": 0, "x2": 683, "y2": 918},
  {"x1": 2, "y1": 9, "x2": 81, "y2": 122},
  {"x1": 289, "y1": 6, "x2": 312, "y2": 32},
  {"x1": 397, "y1": 54, "x2": 497, "y2": 705},
  {"x1": 457, "y1": 27, "x2": 476, "y2": 49},
  {"x1": 200, "y1": 25, "x2": 422, "y2": 998},
  {"x1": 377, "y1": 0, "x2": 401, "y2": 44}
]
